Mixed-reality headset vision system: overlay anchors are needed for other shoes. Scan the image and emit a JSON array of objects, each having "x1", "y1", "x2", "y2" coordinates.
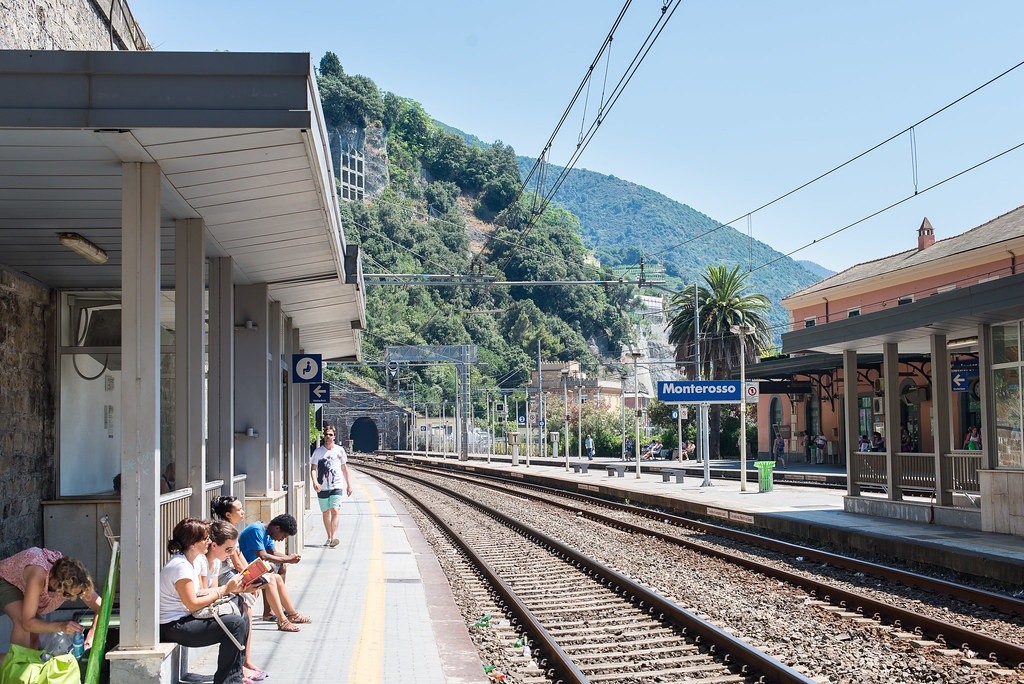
[
  {"x1": 329, "y1": 539, "x2": 339, "y2": 547},
  {"x1": 241, "y1": 677, "x2": 254, "y2": 684},
  {"x1": 249, "y1": 667, "x2": 268, "y2": 680},
  {"x1": 324, "y1": 539, "x2": 331, "y2": 545}
]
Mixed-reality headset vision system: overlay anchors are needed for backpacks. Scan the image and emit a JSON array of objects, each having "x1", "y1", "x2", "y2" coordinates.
[{"x1": 776, "y1": 438, "x2": 784, "y2": 453}]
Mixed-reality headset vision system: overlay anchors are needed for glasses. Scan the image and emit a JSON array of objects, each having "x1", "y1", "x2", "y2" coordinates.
[
  {"x1": 324, "y1": 433, "x2": 333, "y2": 436},
  {"x1": 59, "y1": 583, "x2": 83, "y2": 597},
  {"x1": 221, "y1": 542, "x2": 235, "y2": 554},
  {"x1": 201, "y1": 535, "x2": 210, "y2": 541}
]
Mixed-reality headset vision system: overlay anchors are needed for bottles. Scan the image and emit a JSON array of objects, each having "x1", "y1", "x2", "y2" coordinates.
[{"x1": 74, "y1": 632, "x2": 84, "y2": 657}]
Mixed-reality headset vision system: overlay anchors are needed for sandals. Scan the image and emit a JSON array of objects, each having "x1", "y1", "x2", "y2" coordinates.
[
  {"x1": 278, "y1": 619, "x2": 300, "y2": 631},
  {"x1": 288, "y1": 613, "x2": 311, "y2": 623}
]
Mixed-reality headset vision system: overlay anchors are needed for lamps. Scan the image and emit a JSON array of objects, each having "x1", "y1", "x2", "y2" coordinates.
[{"x1": 55, "y1": 232, "x2": 109, "y2": 264}]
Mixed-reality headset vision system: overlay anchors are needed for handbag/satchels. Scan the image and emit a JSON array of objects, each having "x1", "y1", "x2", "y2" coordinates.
[
  {"x1": 192, "y1": 593, "x2": 241, "y2": 618},
  {"x1": 0, "y1": 643, "x2": 81, "y2": 684},
  {"x1": 816, "y1": 444, "x2": 824, "y2": 449}
]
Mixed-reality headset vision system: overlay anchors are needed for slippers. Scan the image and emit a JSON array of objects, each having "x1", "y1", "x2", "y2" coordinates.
[
  {"x1": 283, "y1": 610, "x2": 289, "y2": 617},
  {"x1": 263, "y1": 614, "x2": 278, "y2": 622}
]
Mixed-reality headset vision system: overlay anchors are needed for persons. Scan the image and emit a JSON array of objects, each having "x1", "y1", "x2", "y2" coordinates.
[
  {"x1": 238, "y1": 513, "x2": 301, "y2": 622},
  {"x1": 161, "y1": 463, "x2": 175, "y2": 495},
  {"x1": 625, "y1": 435, "x2": 634, "y2": 461},
  {"x1": 0, "y1": 547, "x2": 102, "y2": 650},
  {"x1": 675, "y1": 440, "x2": 696, "y2": 461},
  {"x1": 160, "y1": 517, "x2": 269, "y2": 684},
  {"x1": 859, "y1": 432, "x2": 887, "y2": 452},
  {"x1": 113, "y1": 474, "x2": 121, "y2": 496},
  {"x1": 210, "y1": 495, "x2": 311, "y2": 632},
  {"x1": 585, "y1": 434, "x2": 595, "y2": 460},
  {"x1": 640, "y1": 440, "x2": 663, "y2": 460},
  {"x1": 964, "y1": 426, "x2": 982, "y2": 450},
  {"x1": 813, "y1": 430, "x2": 827, "y2": 464},
  {"x1": 900, "y1": 422, "x2": 912, "y2": 453},
  {"x1": 311, "y1": 426, "x2": 352, "y2": 547},
  {"x1": 773, "y1": 433, "x2": 786, "y2": 469},
  {"x1": 801, "y1": 430, "x2": 811, "y2": 463}
]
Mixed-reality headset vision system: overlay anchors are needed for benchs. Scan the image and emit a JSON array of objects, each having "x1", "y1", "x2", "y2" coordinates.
[
  {"x1": 654, "y1": 450, "x2": 662, "y2": 459},
  {"x1": 104, "y1": 633, "x2": 190, "y2": 684}
]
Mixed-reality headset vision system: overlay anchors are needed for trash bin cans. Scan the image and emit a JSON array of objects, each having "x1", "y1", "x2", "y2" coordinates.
[{"x1": 754, "y1": 461, "x2": 776, "y2": 493}]
